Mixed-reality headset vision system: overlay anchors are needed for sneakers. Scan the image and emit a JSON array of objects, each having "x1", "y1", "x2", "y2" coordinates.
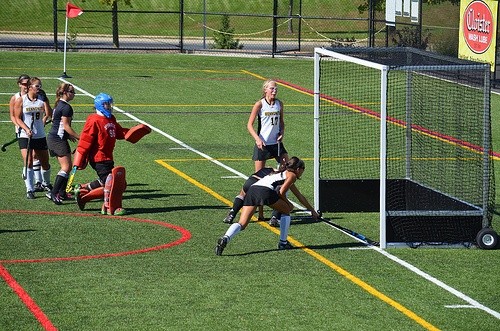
[
  {"x1": 223, "y1": 215, "x2": 233, "y2": 224},
  {"x1": 101, "y1": 204, "x2": 126, "y2": 215},
  {"x1": 41, "y1": 184, "x2": 53, "y2": 192},
  {"x1": 45, "y1": 182, "x2": 86, "y2": 211},
  {"x1": 27, "y1": 189, "x2": 36, "y2": 199},
  {"x1": 215, "y1": 238, "x2": 227, "y2": 256},
  {"x1": 34, "y1": 182, "x2": 44, "y2": 192},
  {"x1": 278, "y1": 241, "x2": 294, "y2": 249},
  {"x1": 269, "y1": 218, "x2": 280, "y2": 227}
]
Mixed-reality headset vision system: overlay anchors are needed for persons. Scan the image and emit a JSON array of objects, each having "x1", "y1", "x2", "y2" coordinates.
[
  {"x1": 13, "y1": 77, "x2": 53, "y2": 199},
  {"x1": 73, "y1": 93, "x2": 152, "y2": 215},
  {"x1": 10, "y1": 74, "x2": 52, "y2": 191},
  {"x1": 46, "y1": 83, "x2": 81, "y2": 205},
  {"x1": 247, "y1": 79, "x2": 289, "y2": 200},
  {"x1": 216, "y1": 156, "x2": 319, "y2": 256},
  {"x1": 223, "y1": 167, "x2": 281, "y2": 227}
]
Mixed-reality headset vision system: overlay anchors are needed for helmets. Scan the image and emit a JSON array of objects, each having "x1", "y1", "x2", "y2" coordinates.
[{"x1": 94, "y1": 93, "x2": 113, "y2": 118}]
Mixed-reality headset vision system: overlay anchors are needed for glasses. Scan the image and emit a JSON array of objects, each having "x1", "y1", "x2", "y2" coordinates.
[
  {"x1": 20, "y1": 83, "x2": 28, "y2": 86},
  {"x1": 30, "y1": 84, "x2": 42, "y2": 88},
  {"x1": 66, "y1": 90, "x2": 75, "y2": 94}
]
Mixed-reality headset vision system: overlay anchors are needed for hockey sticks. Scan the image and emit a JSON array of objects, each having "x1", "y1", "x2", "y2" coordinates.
[
  {"x1": 22, "y1": 117, "x2": 34, "y2": 180},
  {"x1": 276, "y1": 133, "x2": 280, "y2": 170},
  {"x1": 317, "y1": 217, "x2": 380, "y2": 246},
  {"x1": 66, "y1": 166, "x2": 78, "y2": 193},
  {"x1": 290, "y1": 210, "x2": 322, "y2": 220},
  {"x1": 1, "y1": 119, "x2": 52, "y2": 153}
]
